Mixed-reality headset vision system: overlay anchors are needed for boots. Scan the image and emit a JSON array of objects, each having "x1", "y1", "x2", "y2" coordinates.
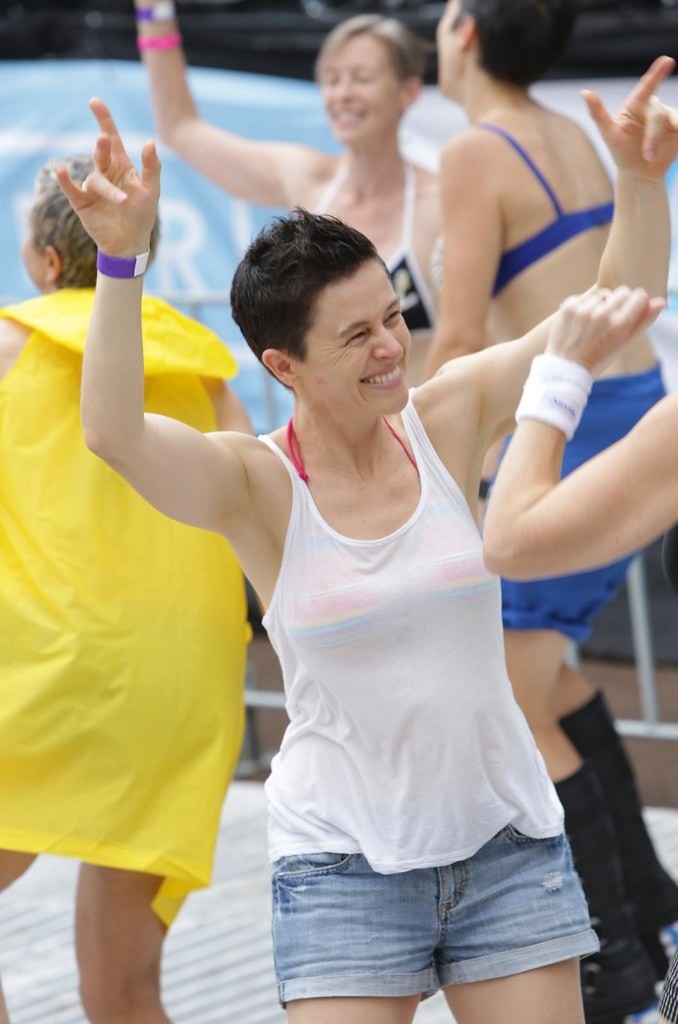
[
  {"x1": 552, "y1": 764, "x2": 659, "y2": 1024},
  {"x1": 556, "y1": 689, "x2": 670, "y2": 980}
]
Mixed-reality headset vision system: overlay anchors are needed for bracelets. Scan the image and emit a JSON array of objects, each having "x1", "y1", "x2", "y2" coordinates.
[
  {"x1": 94, "y1": 250, "x2": 152, "y2": 280},
  {"x1": 133, "y1": 3, "x2": 178, "y2": 22},
  {"x1": 137, "y1": 33, "x2": 183, "y2": 50},
  {"x1": 479, "y1": 479, "x2": 489, "y2": 499},
  {"x1": 513, "y1": 355, "x2": 594, "y2": 440}
]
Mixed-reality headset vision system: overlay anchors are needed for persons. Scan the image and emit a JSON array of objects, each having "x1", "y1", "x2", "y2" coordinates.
[
  {"x1": 423, "y1": 0, "x2": 678, "y2": 1024},
  {"x1": 0, "y1": 143, "x2": 273, "y2": 1024},
  {"x1": 131, "y1": 0, "x2": 443, "y2": 405},
  {"x1": 55, "y1": 53, "x2": 678, "y2": 1024},
  {"x1": 480, "y1": 285, "x2": 678, "y2": 587}
]
